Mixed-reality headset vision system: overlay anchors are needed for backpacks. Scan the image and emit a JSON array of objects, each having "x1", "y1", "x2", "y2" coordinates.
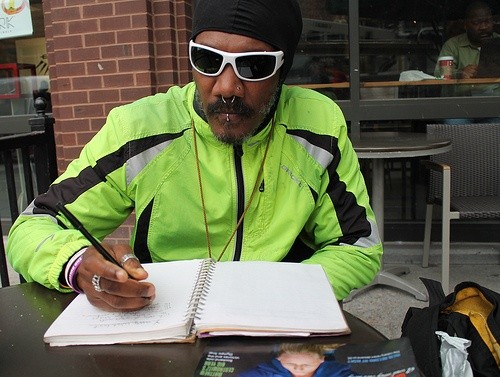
[{"x1": 400, "y1": 278, "x2": 500, "y2": 377}]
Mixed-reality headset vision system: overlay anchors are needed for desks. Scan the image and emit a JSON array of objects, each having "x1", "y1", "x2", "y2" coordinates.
[
  {"x1": 342, "y1": 132, "x2": 453, "y2": 302},
  {"x1": 0, "y1": 280, "x2": 389, "y2": 376},
  {"x1": 287, "y1": 77, "x2": 500, "y2": 90}
]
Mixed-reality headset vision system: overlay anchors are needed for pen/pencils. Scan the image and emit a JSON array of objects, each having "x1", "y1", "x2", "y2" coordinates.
[{"x1": 53, "y1": 201, "x2": 136, "y2": 280}]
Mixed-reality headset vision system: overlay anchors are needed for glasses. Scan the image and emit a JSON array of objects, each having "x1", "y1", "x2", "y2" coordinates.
[{"x1": 189, "y1": 40, "x2": 284, "y2": 82}]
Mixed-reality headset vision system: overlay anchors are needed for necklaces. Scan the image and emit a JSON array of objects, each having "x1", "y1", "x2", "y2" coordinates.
[{"x1": 186, "y1": 107, "x2": 277, "y2": 263}]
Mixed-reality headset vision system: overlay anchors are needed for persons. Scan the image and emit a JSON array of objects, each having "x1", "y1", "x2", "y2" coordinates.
[
  {"x1": 233, "y1": 342, "x2": 359, "y2": 377},
  {"x1": 431, "y1": 0, "x2": 500, "y2": 128},
  {"x1": 13, "y1": 0, "x2": 384, "y2": 312}
]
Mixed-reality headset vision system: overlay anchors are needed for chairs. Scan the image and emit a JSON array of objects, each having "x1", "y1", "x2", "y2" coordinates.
[{"x1": 420, "y1": 123, "x2": 500, "y2": 296}]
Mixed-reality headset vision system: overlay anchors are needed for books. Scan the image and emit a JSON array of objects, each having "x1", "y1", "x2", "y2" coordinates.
[
  {"x1": 191, "y1": 339, "x2": 421, "y2": 377},
  {"x1": 42, "y1": 260, "x2": 352, "y2": 351}
]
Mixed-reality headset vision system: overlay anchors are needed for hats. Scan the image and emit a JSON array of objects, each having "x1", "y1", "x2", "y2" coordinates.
[{"x1": 189, "y1": 0, "x2": 303, "y2": 81}]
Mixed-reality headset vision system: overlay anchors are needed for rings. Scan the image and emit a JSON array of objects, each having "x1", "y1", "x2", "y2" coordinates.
[
  {"x1": 121, "y1": 254, "x2": 140, "y2": 270},
  {"x1": 91, "y1": 274, "x2": 106, "y2": 293}
]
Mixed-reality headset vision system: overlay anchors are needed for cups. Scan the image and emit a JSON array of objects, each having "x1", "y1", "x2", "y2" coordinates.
[{"x1": 438, "y1": 56, "x2": 454, "y2": 80}]
